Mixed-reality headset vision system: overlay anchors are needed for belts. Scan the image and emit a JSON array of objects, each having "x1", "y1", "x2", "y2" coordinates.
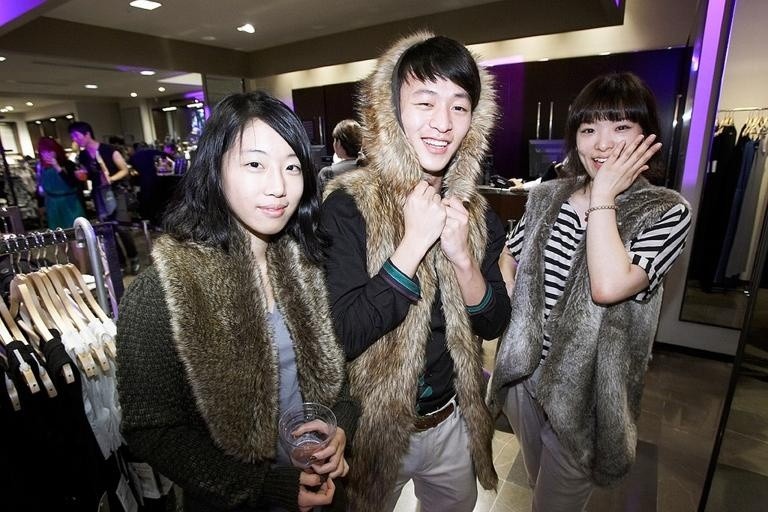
[{"x1": 402, "y1": 400, "x2": 462, "y2": 431}]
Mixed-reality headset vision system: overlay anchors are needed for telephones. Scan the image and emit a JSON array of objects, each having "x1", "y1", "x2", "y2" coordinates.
[{"x1": 488, "y1": 175, "x2": 516, "y2": 189}]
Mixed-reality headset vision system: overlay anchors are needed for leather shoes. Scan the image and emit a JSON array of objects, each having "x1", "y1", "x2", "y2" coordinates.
[{"x1": 130, "y1": 260, "x2": 141, "y2": 274}]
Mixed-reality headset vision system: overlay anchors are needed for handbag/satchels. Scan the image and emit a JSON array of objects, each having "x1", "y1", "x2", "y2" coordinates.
[{"x1": 34, "y1": 161, "x2": 46, "y2": 208}]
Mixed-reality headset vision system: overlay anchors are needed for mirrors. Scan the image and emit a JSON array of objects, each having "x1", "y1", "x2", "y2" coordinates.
[{"x1": 654, "y1": 0, "x2": 767, "y2": 362}]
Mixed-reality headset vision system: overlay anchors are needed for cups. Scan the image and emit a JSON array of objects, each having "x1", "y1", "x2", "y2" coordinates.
[{"x1": 277, "y1": 402, "x2": 338, "y2": 479}]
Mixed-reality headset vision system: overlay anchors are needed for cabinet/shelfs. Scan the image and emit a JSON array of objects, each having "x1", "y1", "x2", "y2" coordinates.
[
  {"x1": 293, "y1": 80, "x2": 361, "y2": 154},
  {"x1": 481, "y1": 45, "x2": 692, "y2": 192}
]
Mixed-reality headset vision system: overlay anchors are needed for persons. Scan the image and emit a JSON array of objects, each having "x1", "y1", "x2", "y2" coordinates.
[
  {"x1": 314, "y1": 119, "x2": 367, "y2": 204},
  {"x1": 150, "y1": 131, "x2": 202, "y2": 175},
  {"x1": 111, "y1": 88, "x2": 366, "y2": 512},
  {"x1": 316, "y1": 25, "x2": 514, "y2": 512},
  {"x1": 126, "y1": 142, "x2": 173, "y2": 234},
  {"x1": 37, "y1": 136, "x2": 92, "y2": 276},
  {"x1": 68, "y1": 121, "x2": 141, "y2": 278},
  {"x1": 480, "y1": 70, "x2": 693, "y2": 512}
]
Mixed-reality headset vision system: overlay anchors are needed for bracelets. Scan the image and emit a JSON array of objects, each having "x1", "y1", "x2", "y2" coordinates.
[{"x1": 583, "y1": 205, "x2": 618, "y2": 214}]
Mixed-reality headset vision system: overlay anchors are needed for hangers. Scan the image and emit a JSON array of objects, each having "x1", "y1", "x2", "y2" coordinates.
[
  {"x1": 711, "y1": 108, "x2": 767, "y2": 141},
  {"x1": 0, "y1": 228, "x2": 116, "y2": 411}
]
